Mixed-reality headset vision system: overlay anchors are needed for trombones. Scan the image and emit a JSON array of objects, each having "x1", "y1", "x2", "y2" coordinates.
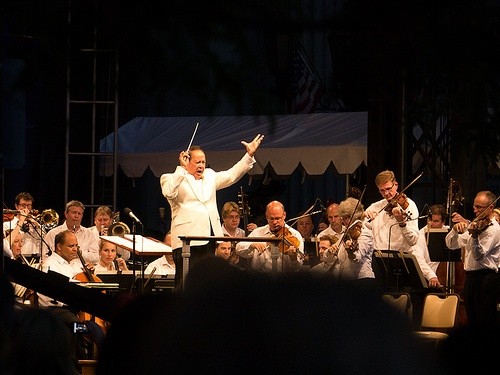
[
  {"x1": 27, "y1": 209, "x2": 60, "y2": 229},
  {"x1": 106, "y1": 212, "x2": 131, "y2": 236}
]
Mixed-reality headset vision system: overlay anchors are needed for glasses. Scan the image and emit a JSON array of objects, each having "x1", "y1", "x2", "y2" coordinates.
[
  {"x1": 225, "y1": 216, "x2": 241, "y2": 220},
  {"x1": 378, "y1": 184, "x2": 394, "y2": 194},
  {"x1": 268, "y1": 215, "x2": 283, "y2": 222},
  {"x1": 473, "y1": 206, "x2": 488, "y2": 211},
  {"x1": 18, "y1": 204, "x2": 32, "y2": 209}
]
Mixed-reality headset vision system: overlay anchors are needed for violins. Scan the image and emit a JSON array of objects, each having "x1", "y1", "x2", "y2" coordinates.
[
  {"x1": 343, "y1": 221, "x2": 362, "y2": 260},
  {"x1": 385, "y1": 193, "x2": 413, "y2": 220},
  {"x1": 468, "y1": 216, "x2": 491, "y2": 235},
  {"x1": 273, "y1": 227, "x2": 309, "y2": 262},
  {"x1": 3, "y1": 208, "x2": 15, "y2": 222}
]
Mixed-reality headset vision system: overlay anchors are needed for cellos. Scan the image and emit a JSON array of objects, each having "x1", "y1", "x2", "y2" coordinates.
[
  {"x1": 446, "y1": 178, "x2": 464, "y2": 226},
  {"x1": 19, "y1": 252, "x2": 39, "y2": 306},
  {"x1": 315, "y1": 187, "x2": 362, "y2": 234},
  {"x1": 237, "y1": 186, "x2": 251, "y2": 238},
  {"x1": 72, "y1": 246, "x2": 108, "y2": 341}
]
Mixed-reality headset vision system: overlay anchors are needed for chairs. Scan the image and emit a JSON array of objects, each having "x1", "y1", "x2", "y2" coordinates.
[
  {"x1": 414, "y1": 291, "x2": 461, "y2": 349},
  {"x1": 384, "y1": 290, "x2": 414, "y2": 323}
]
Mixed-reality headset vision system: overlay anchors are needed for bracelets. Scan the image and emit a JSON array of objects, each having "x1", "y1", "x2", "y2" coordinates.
[
  {"x1": 399, "y1": 223, "x2": 406, "y2": 227},
  {"x1": 467, "y1": 220, "x2": 471, "y2": 224}
]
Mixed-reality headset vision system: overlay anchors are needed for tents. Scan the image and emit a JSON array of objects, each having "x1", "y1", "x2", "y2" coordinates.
[{"x1": 100, "y1": 111, "x2": 369, "y2": 200}]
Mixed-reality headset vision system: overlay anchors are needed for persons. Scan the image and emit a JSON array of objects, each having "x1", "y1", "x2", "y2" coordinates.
[
  {"x1": 160, "y1": 134, "x2": 265, "y2": 287},
  {"x1": 363, "y1": 169, "x2": 420, "y2": 254},
  {"x1": 213, "y1": 201, "x2": 347, "y2": 275},
  {"x1": 413, "y1": 204, "x2": 471, "y2": 289},
  {"x1": 323, "y1": 197, "x2": 376, "y2": 280},
  {"x1": 446, "y1": 191, "x2": 500, "y2": 321},
  {"x1": 0, "y1": 192, "x2": 176, "y2": 375}
]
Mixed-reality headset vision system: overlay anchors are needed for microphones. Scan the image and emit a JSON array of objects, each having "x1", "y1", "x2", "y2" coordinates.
[
  {"x1": 3, "y1": 208, "x2": 21, "y2": 213},
  {"x1": 123, "y1": 208, "x2": 142, "y2": 224},
  {"x1": 319, "y1": 200, "x2": 328, "y2": 211},
  {"x1": 427, "y1": 207, "x2": 433, "y2": 219}
]
